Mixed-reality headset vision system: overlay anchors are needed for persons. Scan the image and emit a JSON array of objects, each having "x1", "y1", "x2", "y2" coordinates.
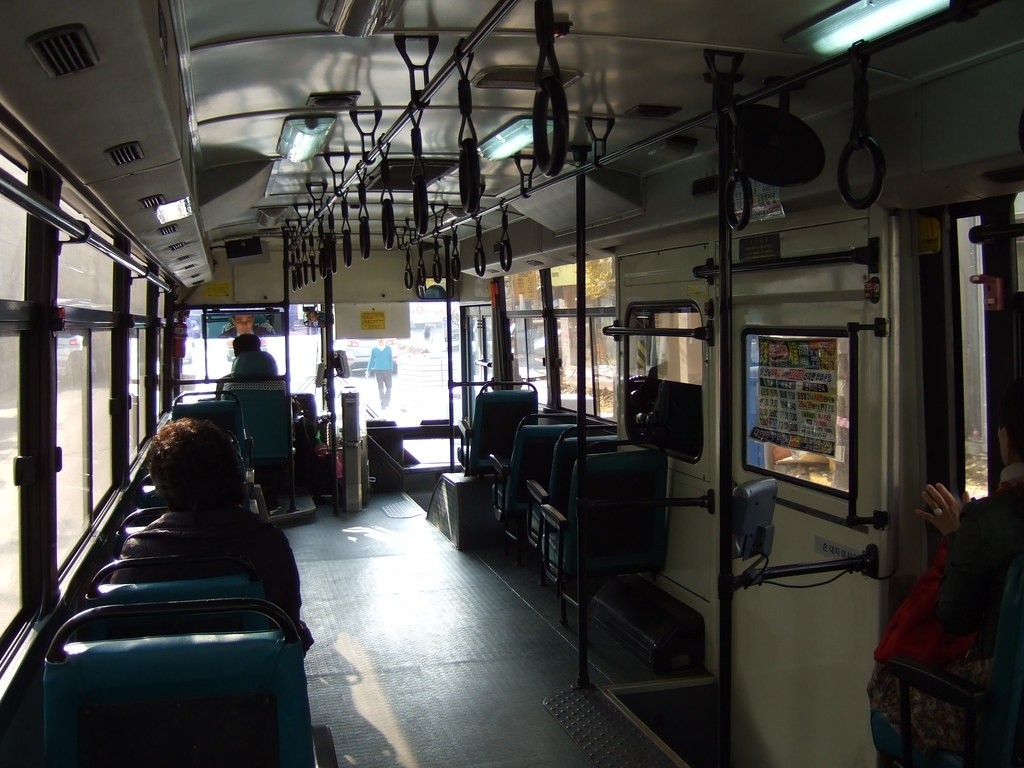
[
  {"x1": 868, "y1": 376, "x2": 1024, "y2": 768},
  {"x1": 304, "y1": 311, "x2": 320, "y2": 326},
  {"x1": 366, "y1": 338, "x2": 394, "y2": 409},
  {"x1": 215, "y1": 333, "x2": 262, "y2": 400},
  {"x1": 111, "y1": 417, "x2": 315, "y2": 655},
  {"x1": 217, "y1": 314, "x2": 274, "y2": 336}
]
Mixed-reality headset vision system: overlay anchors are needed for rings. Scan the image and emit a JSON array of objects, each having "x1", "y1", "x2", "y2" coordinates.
[{"x1": 933, "y1": 508, "x2": 942, "y2": 516}]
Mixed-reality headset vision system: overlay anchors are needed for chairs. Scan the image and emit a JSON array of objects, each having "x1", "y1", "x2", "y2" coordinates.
[
  {"x1": 464, "y1": 381, "x2": 667, "y2": 624},
  {"x1": 42, "y1": 381, "x2": 341, "y2": 767}
]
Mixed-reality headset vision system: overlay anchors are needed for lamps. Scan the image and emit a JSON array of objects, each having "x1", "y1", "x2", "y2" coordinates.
[
  {"x1": 276, "y1": 118, "x2": 336, "y2": 165},
  {"x1": 477, "y1": 118, "x2": 553, "y2": 162},
  {"x1": 781, "y1": 0, "x2": 954, "y2": 59}
]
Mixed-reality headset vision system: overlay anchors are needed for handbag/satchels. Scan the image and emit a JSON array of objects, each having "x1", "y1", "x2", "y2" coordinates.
[{"x1": 873, "y1": 537, "x2": 979, "y2": 669}]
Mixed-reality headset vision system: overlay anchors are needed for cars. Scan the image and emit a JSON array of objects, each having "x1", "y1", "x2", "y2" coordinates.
[
  {"x1": 332, "y1": 339, "x2": 398, "y2": 376},
  {"x1": 57, "y1": 333, "x2": 84, "y2": 391},
  {"x1": 227, "y1": 337, "x2": 269, "y2": 363},
  {"x1": 183, "y1": 315, "x2": 202, "y2": 364}
]
